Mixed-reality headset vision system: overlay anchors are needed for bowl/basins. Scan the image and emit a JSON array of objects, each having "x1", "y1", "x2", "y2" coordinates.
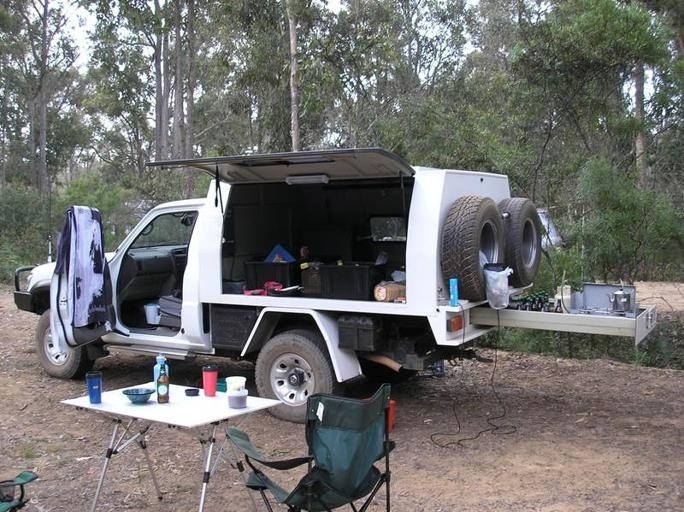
[{"x1": 121, "y1": 387, "x2": 156, "y2": 403}]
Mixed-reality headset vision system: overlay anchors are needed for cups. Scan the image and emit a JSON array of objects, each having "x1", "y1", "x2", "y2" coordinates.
[
  {"x1": 202, "y1": 364, "x2": 218, "y2": 397},
  {"x1": 224, "y1": 390, "x2": 247, "y2": 408},
  {"x1": 85, "y1": 370, "x2": 103, "y2": 405},
  {"x1": 224, "y1": 375, "x2": 246, "y2": 391}
]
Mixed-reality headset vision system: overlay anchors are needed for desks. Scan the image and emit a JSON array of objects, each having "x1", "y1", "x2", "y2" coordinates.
[{"x1": 60, "y1": 381, "x2": 284, "y2": 512}]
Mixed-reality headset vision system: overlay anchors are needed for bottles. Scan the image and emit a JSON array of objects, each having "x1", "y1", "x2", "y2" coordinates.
[
  {"x1": 156, "y1": 363, "x2": 169, "y2": 403},
  {"x1": 152, "y1": 356, "x2": 168, "y2": 389},
  {"x1": 512, "y1": 290, "x2": 563, "y2": 313}
]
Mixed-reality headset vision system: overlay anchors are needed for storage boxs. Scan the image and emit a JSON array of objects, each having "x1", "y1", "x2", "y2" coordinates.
[
  {"x1": 242, "y1": 256, "x2": 314, "y2": 296},
  {"x1": 320, "y1": 260, "x2": 391, "y2": 301}
]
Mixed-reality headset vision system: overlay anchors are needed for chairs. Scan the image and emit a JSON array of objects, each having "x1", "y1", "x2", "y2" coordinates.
[
  {"x1": 225, "y1": 384, "x2": 395, "y2": 512},
  {"x1": 158, "y1": 290, "x2": 181, "y2": 328}
]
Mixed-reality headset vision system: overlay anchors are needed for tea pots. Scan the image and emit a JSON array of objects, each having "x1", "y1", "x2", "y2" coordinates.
[{"x1": 605, "y1": 288, "x2": 630, "y2": 312}]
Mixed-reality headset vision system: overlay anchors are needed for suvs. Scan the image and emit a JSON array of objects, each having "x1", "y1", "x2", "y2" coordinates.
[{"x1": 11, "y1": 146, "x2": 542, "y2": 419}]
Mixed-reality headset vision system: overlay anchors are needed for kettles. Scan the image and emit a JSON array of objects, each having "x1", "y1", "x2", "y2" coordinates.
[{"x1": 143, "y1": 302, "x2": 161, "y2": 325}]
